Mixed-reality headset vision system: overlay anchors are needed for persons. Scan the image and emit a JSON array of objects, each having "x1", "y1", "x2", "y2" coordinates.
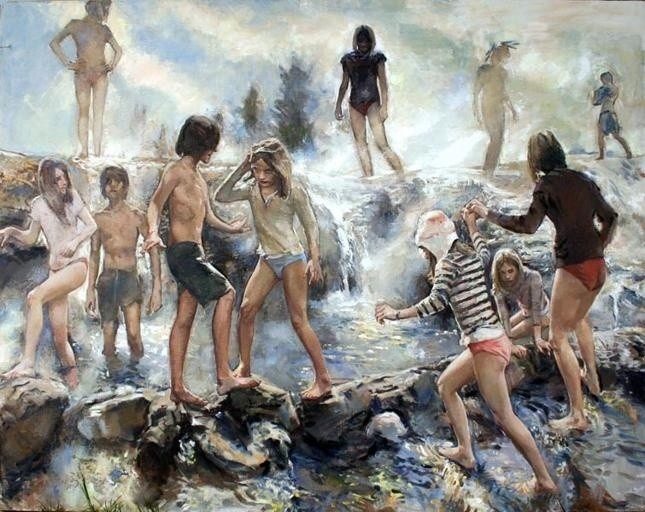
[
  {"x1": 331, "y1": 22, "x2": 404, "y2": 176},
  {"x1": 590, "y1": 67, "x2": 633, "y2": 161},
  {"x1": 1, "y1": 155, "x2": 100, "y2": 389},
  {"x1": 84, "y1": 163, "x2": 165, "y2": 358},
  {"x1": 489, "y1": 246, "x2": 555, "y2": 360},
  {"x1": 214, "y1": 137, "x2": 335, "y2": 402},
  {"x1": 374, "y1": 204, "x2": 558, "y2": 497},
  {"x1": 48, "y1": 1, "x2": 125, "y2": 162},
  {"x1": 471, "y1": 38, "x2": 520, "y2": 181},
  {"x1": 467, "y1": 127, "x2": 621, "y2": 435},
  {"x1": 140, "y1": 110, "x2": 262, "y2": 407}
]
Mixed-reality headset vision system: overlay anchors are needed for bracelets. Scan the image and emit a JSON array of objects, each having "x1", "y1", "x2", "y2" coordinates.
[{"x1": 393, "y1": 308, "x2": 402, "y2": 322}]
